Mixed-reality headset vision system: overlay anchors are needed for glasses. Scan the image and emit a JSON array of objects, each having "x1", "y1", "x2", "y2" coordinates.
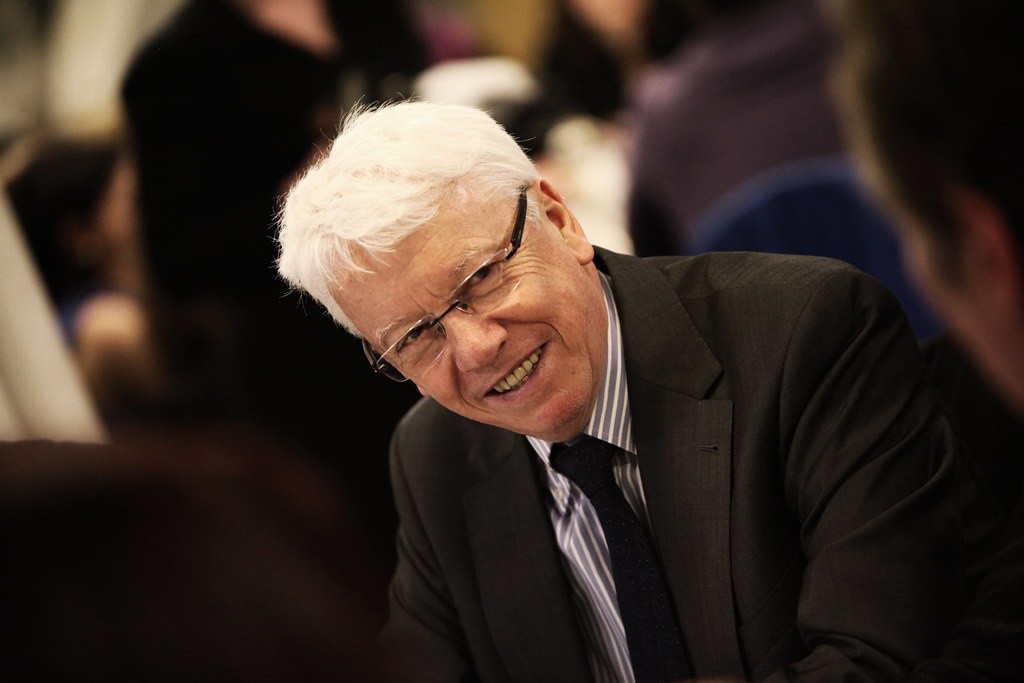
[{"x1": 361, "y1": 185, "x2": 529, "y2": 382}]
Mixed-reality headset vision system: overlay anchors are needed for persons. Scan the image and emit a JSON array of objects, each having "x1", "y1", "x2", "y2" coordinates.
[
  {"x1": 505, "y1": 0, "x2": 649, "y2": 162},
  {"x1": 0, "y1": 131, "x2": 164, "y2": 444},
  {"x1": 119, "y1": 0, "x2": 442, "y2": 576},
  {"x1": 819, "y1": 0, "x2": 1024, "y2": 407},
  {"x1": 279, "y1": 94, "x2": 1024, "y2": 682},
  {"x1": 628, "y1": 0, "x2": 846, "y2": 257}
]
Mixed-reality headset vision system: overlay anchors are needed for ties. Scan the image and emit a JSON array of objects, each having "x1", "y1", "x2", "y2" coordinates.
[{"x1": 549, "y1": 435, "x2": 692, "y2": 683}]
